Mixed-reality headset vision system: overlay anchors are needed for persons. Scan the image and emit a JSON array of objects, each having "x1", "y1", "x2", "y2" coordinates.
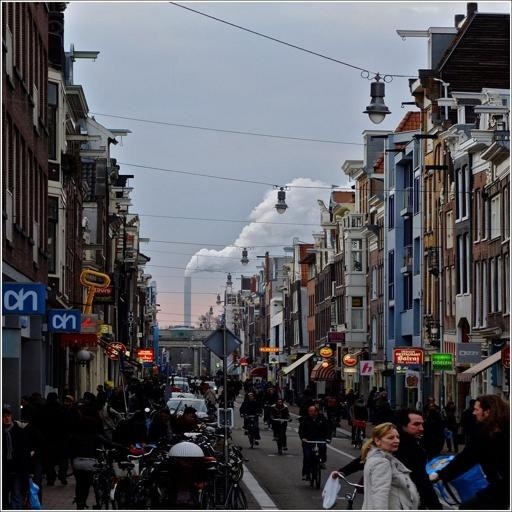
[
  {"x1": 214, "y1": 369, "x2": 509, "y2": 511},
  {"x1": 2, "y1": 369, "x2": 175, "y2": 511}
]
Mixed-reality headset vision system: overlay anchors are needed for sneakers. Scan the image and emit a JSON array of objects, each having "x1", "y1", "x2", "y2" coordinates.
[
  {"x1": 244, "y1": 431, "x2": 248, "y2": 436},
  {"x1": 255, "y1": 441, "x2": 259, "y2": 445},
  {"x1": 301, "y1": 476, "x2": 307, "y2": 481},
  {"x1": 321, "y1": 463, "x2": 327, "y2": 470}
]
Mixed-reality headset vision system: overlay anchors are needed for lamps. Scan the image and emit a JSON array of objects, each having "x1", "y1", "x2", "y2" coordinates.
[
  {"x1": 359, "y1": 69, "x2": 393, "y2": 124},
  {"x1": 74, "y1": 345, "x2": 93, "y2": 367},
  {"x1": 207, "y1": 246, "x2": 250, "y2": 316},
  {"x1": 273, "y1": 185, "x2": 291, "y2": 214}
]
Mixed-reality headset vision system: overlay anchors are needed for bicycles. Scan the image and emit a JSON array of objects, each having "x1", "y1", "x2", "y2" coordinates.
[
  {"x1": 333, "y1": 471, "x2": 365, "y2": 509},
  {"x1": 300, "y1": 437, "x2": 327, "y2": 487},
  {"x1": 239, "y1": 412, "x2": 262, "y2": 448},
  {"x1": 273, "y1": 418, "x2": 291, "y2": 453},
  {"x1": 90, "y1": 412, "x2": 249, "y2": 509},
  {"x1": 351, "y1": 420, "x2": 368, "y2": 450},
  {"x1": 263, "y1": 403, "x2": 276, "y2": 429}
]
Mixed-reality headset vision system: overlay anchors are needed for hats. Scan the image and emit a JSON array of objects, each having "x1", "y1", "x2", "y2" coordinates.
[{"x1": 3, "y1": 402, "x2": 13, "y2": 414}]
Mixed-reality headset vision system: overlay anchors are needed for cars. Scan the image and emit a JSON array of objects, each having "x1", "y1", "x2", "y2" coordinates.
[{"x1": 166, "y1": 371, "x2": 219, "y2": 421}]
